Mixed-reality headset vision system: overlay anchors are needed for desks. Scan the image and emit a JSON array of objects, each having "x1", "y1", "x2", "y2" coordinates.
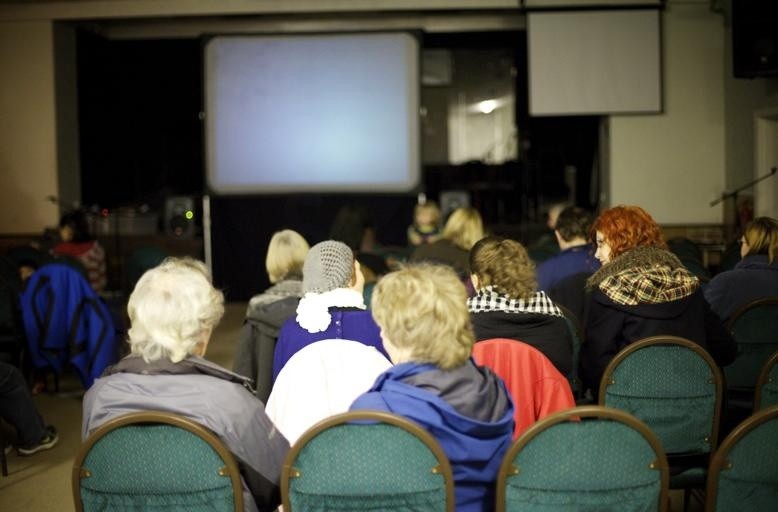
[{"x1": 95, "y1": 232, "x2": 202, "y2": 294}]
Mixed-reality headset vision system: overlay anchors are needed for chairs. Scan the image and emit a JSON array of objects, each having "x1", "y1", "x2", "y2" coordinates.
[
  {"x1": 721, "y1": 296, "x2": 778, "y2": 401},
  {"x1": 494, "y1": 405, "x2": 668, "y2": 511},
  {"x1": 264, "y1": 339, "x2": 395, "y2": 448},
  {"x1": 469, "y1": 338, "x2": 580, "y2": 442},
  {"x1": 22, "y1": 265, "x2": 109, "y2": 395},
  {"x1": 71, "y1": 411, "x2": 245, "y2": 511},
  {"x1": 279, "y1": 410, "x2": 455, "y2": 512},
  {"x1": 752, "y1": 351, "x2": 778, "y2": 410},
  {"x1": 598, "y1": 335, "x2": 723, "y2": 512},
  {"x1": 703, "y1": 405, "x2": 778, "y2": 511}
]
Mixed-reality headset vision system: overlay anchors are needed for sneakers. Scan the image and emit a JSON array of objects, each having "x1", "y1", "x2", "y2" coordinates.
[
  {"x1": 4, "y1": 431, "x2": 17, "y2": 455},
  {"x1": 18, "y1": 424, "x2": 61, "y2": 456}
]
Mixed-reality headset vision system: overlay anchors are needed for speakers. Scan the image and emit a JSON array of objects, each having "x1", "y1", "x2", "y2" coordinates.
[
  {"x1": 440, "y1": 191, "x2": 470, "y2": 227},
  {"x1": 164, "y1": 196, "x2": 195, "y2": 240}
]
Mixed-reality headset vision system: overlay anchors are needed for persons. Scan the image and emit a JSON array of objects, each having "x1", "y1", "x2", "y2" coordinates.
[{"x1": 0, "y1": 200, "x2": 778, "y2": 512}]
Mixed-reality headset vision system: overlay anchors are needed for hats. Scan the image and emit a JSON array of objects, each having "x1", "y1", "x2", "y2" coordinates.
[{"x1": 302, "y1": 239, "x2": 354, "y2": 294}]
